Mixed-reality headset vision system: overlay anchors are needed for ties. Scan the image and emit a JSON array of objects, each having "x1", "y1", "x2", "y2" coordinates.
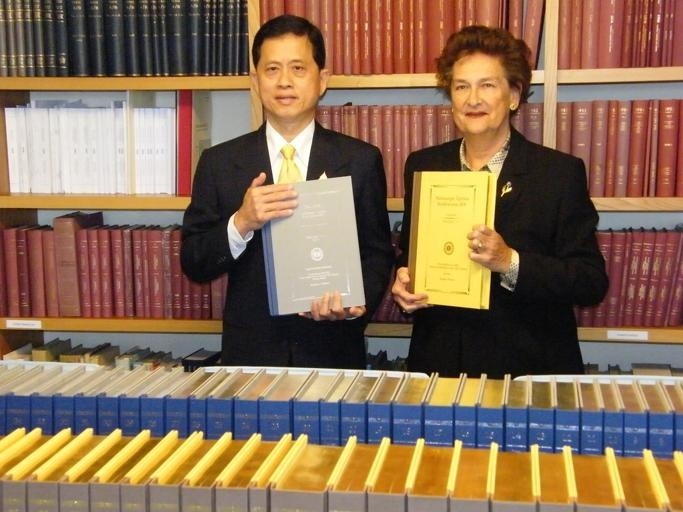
[{"x1": 278, "y1": 146, "x2": 302, "y2": 184}]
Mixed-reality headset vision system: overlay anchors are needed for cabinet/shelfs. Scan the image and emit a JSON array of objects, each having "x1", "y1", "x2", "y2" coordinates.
[{"x1": 0, "y1": 0, "x2": 683, "y2": 363}]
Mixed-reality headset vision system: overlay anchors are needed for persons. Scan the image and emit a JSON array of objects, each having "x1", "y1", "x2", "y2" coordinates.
[
  {"x1": 391, "y1": 26, "x2": 609, "y2": 378},
  {"x1": 179, "y1": 14, "x2": 396, "y2": 369}
]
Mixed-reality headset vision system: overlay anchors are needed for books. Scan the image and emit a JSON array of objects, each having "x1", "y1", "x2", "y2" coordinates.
[
  {"x1": 1, "y1": 0, "x2": 250, "y2": 76},
  {"x1": 408, "y1": 171, "x2": 498, "y2": 310},
  {"x1": 1, "y1": 212, "x2": 227, "y2": 320},
  {"x1": 575, "y1": 224, "x2": 683, "y2": 328},
  {"x1": 5, "y1": 106, "x2": 176, "y2": 193},
  {"x1": 1, "y1": 426, "x2": 682, "y2": 512},
  {"x1": 1, "y1": 362, "x2": 683, "y2": 457},
  {"x1": 258, "y1": 176, "x2": 366, "y2": 317},
  {"x1": 556, "y1": 100, "x2": 683, "y2": 202},
  {"x1": 558, "y1": 0, "x2": 683, "y2": 69},
  {"x1": 260, "y1": 1, "x2": 543, "y2": 75},
  {"x1": 315, "y1": 104, "x2": 542, "y2": 198},
  {"x1": 2, "y1": 337, "x2": 221, "y2": 373}
]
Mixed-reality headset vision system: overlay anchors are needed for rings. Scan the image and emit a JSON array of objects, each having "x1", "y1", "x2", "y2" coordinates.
[{"x1": 471, "y1": 239, "x2": 484, "y2": 252}]
